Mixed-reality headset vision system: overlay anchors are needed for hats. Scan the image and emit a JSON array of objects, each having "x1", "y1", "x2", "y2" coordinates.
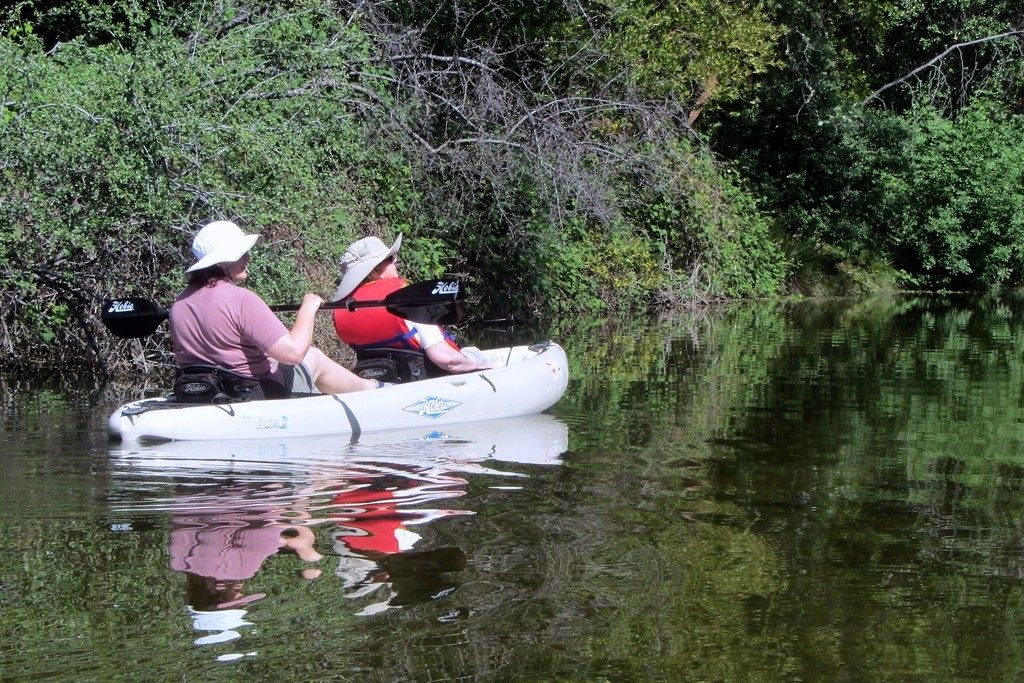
[
  {"x1": 332, "y1": 232, "x2": 403, "y2": 303},
  {"x1": 184, "y1": 220, "x2": 263, "y2": 273}
]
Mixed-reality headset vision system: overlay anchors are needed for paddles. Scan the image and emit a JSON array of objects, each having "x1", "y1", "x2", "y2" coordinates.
[{"x1": 102, "y1": 274, "x2": 466, "y2": 338}]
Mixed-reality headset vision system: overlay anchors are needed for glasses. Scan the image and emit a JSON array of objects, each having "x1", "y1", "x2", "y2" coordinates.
[{"x1": 383, "y1": 255, "x2": 396, "y2": 264}]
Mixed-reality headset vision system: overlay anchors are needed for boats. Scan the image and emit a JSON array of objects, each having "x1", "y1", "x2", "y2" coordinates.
[
  {"x1": 110, "y1": 342, "x2": 568, "y2": 441},
  {"x1": 106, "y1": 418, "x2": 569, "y2": 470}
]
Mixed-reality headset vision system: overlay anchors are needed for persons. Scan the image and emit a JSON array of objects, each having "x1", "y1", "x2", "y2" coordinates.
[
  {"x1": 169, "y1": 221, "x2": 391, "y2": 393},
  {"x1": 333, "y1": 232, "x2": 492, "y2": 373}
]
